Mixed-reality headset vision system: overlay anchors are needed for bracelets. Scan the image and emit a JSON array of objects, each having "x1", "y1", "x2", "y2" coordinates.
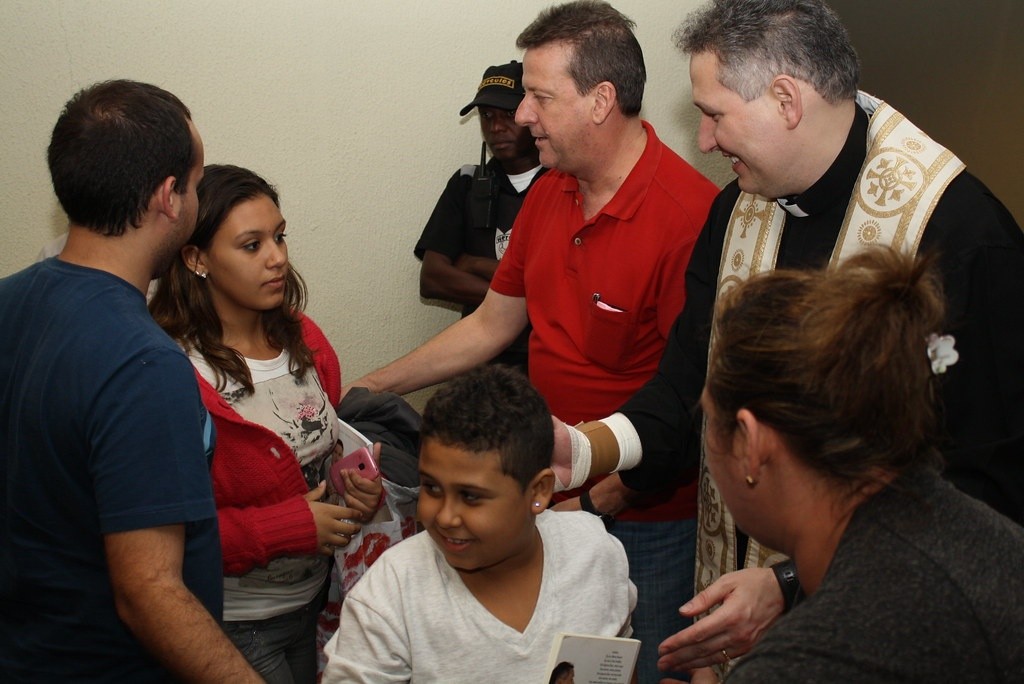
[{"x1": 771, "y1": 559, "x2": 804, "y2": 613}]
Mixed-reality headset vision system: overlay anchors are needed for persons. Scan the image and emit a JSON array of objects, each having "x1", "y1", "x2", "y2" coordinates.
[
  {"x1": 701, "y1": 248, "x2": 1024, "y2": 684},
  {"x1": 549, "y1": 0, "x2": 1024, "y2": 677},
  {"x1": 322, "y1": 365, "x2": 638, "y2": 684},
  {"x1": 151, "y1": 164, "x2": 384, "y2": 683},
  {"x1": 325, "y1": 439, "x2": 344, "y2": 498},
  {"x1": 416, "y1": 61, "x2": 534, "y2": 377},
  {"x1": 0, "y1": 79, "x2": 266, "y2": 684},
  {"x1": 334, "y1": 2, "x2": 721, "y2": 684},
  {"x1": 549, "y1": 662, "x2": 575, "y2": 684}
]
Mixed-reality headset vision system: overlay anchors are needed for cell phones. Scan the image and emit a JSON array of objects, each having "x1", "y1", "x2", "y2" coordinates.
[{"x1": 329, "y1": 447, "x2": 380, "y2": 497}]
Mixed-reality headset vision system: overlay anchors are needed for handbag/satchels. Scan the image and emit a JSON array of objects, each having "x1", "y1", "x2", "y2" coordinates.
[{"x1": 316, "y1": 416, "x2": 422, "y2": 684}]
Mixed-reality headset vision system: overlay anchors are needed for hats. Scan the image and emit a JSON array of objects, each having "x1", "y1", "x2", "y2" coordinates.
[{"x1": 460, "y1": 60, "x2": 524, "y2": 117}]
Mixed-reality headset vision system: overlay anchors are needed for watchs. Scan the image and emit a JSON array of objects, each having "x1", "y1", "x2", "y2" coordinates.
[{"x1": 580, "y1": 491, "x2": 614, "y2": 525}]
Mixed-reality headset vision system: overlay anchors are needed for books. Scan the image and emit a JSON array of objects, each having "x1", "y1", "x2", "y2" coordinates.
[
  {"x1": 324, "y1": 416, "x2": 374, "y2": 511},
  {"x1": 544, "y1": 633, "x2": 641, "y2": 684}
]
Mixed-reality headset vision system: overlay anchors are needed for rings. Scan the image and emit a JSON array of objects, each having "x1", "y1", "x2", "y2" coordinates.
[{"x1": 722, "y1": 650, "x2": 732, "y2": 661}]
